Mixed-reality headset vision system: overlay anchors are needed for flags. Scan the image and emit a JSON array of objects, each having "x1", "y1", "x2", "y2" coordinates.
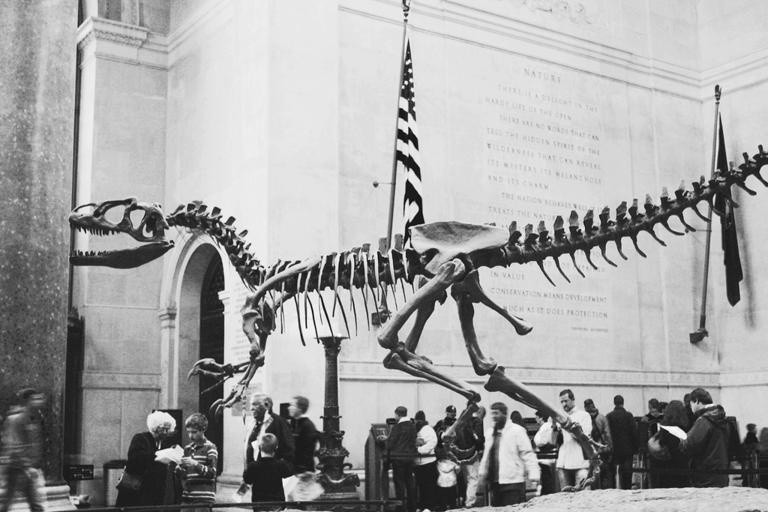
[
  {"x1": 397, "y1": 36, "x2": 424, "y2": 246},
  {"x1": 714, "y1": 113, "x2": 743, "y2": 307}
]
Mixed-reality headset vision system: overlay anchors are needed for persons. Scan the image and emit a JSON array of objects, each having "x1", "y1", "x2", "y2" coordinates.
[
  {"x1": 1, "y1": 386, "x2": 53, "y2": 510},
  {"x1": 531, "y1": 387, "x2": 740, "y2": 491},
  {"x1": 111, "y1": 411, "x2": 177, "y2": 506},
  {"x1": 743, "y1": 423, "x2": 768, "y2": 489},
  {"x1": 176, "y1": 410, "x2": 222, "y2": 511},
  {"x1": 241, "y1": 391, "x2": 316, "y2": 512},
  {"x1": 376, "y1": 399, "x2": 541, "y2": 512}
]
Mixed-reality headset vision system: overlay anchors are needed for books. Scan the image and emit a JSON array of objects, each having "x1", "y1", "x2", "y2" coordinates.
[{"x1": 154, "y1": 448, "x2": 181, "y2": 465}]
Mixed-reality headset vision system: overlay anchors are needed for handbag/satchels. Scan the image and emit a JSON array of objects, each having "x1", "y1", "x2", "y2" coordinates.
[
  {"x1": 538, "y1": 445, "x2": 558, "y2": 459},
  {"x1": 116, "y1": 465, "x2": 146, "y2": 496}
]
